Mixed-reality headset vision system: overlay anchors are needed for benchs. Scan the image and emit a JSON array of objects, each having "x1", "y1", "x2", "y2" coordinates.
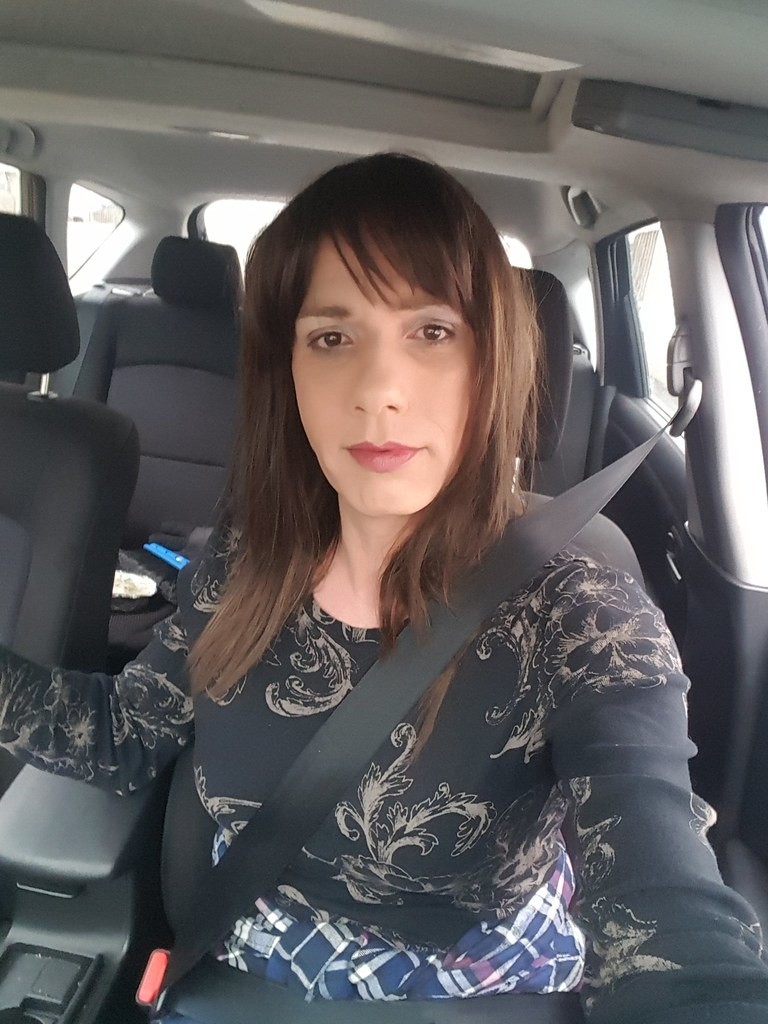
[{"x1": 66, "y1": 234, "x2": 595, "y2": 659}]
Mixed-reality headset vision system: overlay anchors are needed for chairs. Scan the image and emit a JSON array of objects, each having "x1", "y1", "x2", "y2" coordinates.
[
  {"x1": 1, "y1": 210, "x2": 142, "y2": 794},
  {"x1": 497, "y1": 271, "x2": 652, "y2": 598}
]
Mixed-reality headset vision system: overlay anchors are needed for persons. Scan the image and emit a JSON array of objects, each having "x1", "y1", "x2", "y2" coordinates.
[{"x1": 0, "y1": 150, "x2": 768, "y2": 1024}]
